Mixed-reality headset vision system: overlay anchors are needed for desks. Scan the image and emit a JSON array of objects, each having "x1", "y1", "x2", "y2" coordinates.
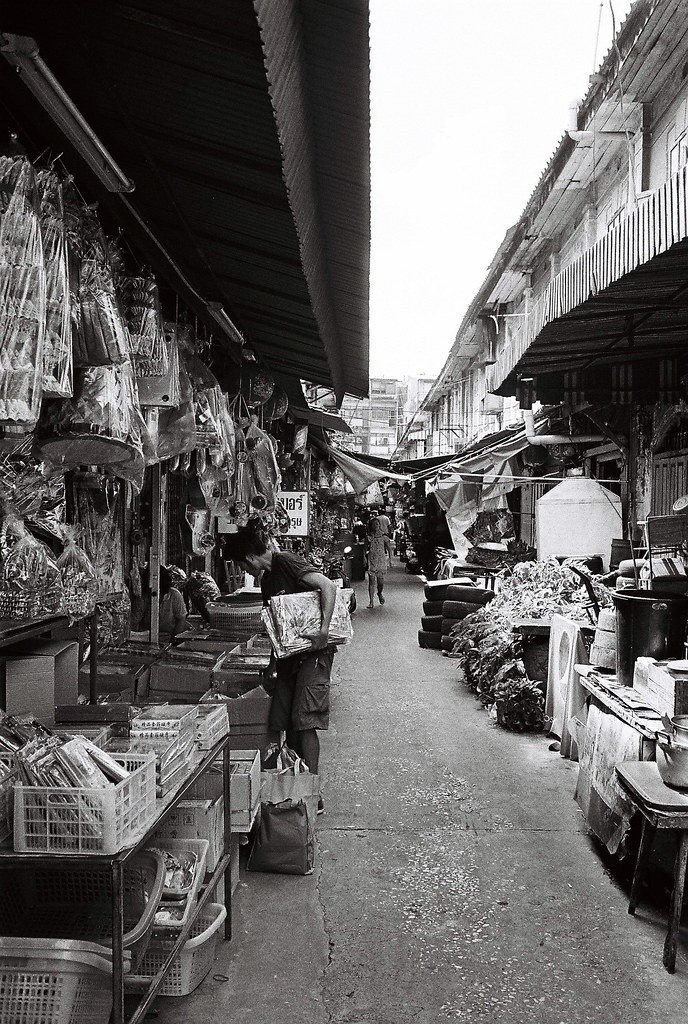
[{"x1": 613, "y1": 759, "x2": 687, "y2": 975}]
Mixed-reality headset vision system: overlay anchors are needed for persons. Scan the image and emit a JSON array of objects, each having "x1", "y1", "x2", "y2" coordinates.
[
  {"x1": 364, "y1": 508, "x2": 395, "y2": 608},
  {"x1": 226, "y1": 525, "x2": 332, "y2": 816},
  {"x1": 143, "y1": 564, "x2": 188, "y2": 637}
]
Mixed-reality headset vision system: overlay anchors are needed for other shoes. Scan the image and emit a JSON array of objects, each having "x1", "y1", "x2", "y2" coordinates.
[{"x1": 318, "y1": 795, "x2": 326, "y2": 814}]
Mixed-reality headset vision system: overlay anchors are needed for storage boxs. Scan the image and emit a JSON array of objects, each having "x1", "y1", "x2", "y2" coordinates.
[
  {"x1": 634, "y1": 658, "x2": 688, "y2": 719},
  {"x1": 0, "y1": 586, "x2": 321, "y2": 1023}
]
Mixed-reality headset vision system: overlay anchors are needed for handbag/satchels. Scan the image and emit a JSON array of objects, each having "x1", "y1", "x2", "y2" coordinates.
[
  {"x1": 244, "y1": 797, "x2": 317, "y2": 875},
  {"x1": 261, "y1": 746, "x2": 321, "y2": 834}
]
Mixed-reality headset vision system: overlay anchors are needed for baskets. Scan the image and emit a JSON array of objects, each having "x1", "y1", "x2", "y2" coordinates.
[
  {"x1": 11, "y1": 752, "x2": 158, "y2": 856},
  {"x1": 149, "y1": 837, "x2": 210, "y2": 928},
  {"x1": 205, "y1": 601, "x2": 266, "y2": 634},
  {"x1": 0, "y1": 751, "x2": 19, "y2": 842},
  {"x1": 0, "y1": 851, "x2": 166, "y2": 975},
  {"x1": 0, "y1": 938, "x2": 132, "y2": 1023},
  {"x1": 122, "y1": 902, "x2": 228, "y2": 996},
  {"x1": 50, "y1": 729, "x2": 108, "y2": 748},
  {"x1": 0, "y1": 539, "x2": 62, "y2": 620}
]
